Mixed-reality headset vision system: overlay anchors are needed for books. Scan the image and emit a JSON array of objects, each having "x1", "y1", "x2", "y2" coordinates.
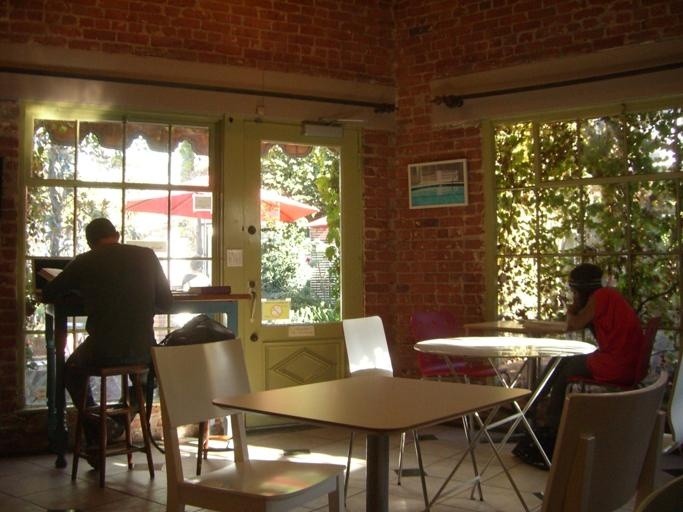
[
  {"x1": 187, "y1": 286, "x2": 231, "y2": 295},
  {"x1": 522, "y1": 319, "x2": 568, "y2": 332},
  {"x1": 35, "y1": 267, "x2": 63, "y2": 282}
]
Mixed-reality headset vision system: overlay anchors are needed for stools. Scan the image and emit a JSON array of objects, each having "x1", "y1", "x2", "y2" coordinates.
[{"x1": 61, "y1": 358, "x2": 155, "y2": 490}]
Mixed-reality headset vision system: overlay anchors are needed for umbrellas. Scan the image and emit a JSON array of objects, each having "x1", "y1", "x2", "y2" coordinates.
[{"x1": 121, "y1": 177, "x2": 317, "y2": 227}]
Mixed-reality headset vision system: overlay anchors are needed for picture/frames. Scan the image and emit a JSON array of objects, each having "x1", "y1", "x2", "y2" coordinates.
[{"x1": 407, "y1": 159, "x2": 468, "y2": 210}]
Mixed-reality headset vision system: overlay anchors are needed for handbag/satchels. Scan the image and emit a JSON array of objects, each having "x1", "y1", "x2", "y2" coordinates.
[{"x1": 165, "y1": 316, "x2": 234, "y2": 346}]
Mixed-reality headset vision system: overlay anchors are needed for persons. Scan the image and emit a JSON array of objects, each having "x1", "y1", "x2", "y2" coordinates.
[
  {"x1": 180, "y1": 255, "x2": 211, "y2": 293},
  {"x1": 38, "y1": 218, "x2": 173, "y2": 467},
  {"x1": 511, "y1": 263, "x2": 647, "y2": 469}
]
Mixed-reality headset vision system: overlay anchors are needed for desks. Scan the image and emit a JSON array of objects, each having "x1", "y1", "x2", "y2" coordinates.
[{"x1": 36, "y1": 286, "x2": 258, "y2": 470}]
